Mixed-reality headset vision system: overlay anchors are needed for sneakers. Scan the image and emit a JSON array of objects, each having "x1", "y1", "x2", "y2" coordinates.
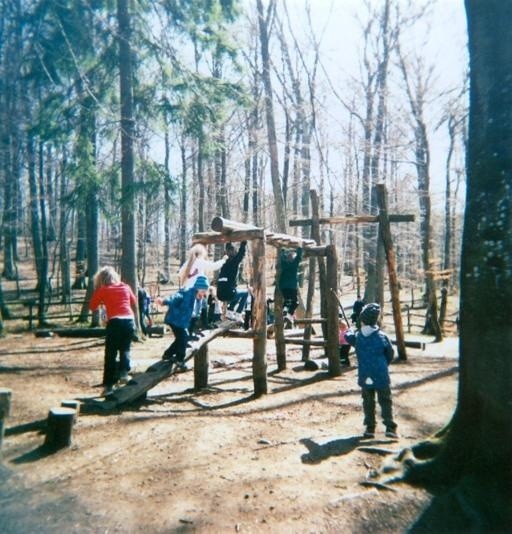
[
  {"x1": 225, "y1": 310, "x2": 236, "y2": 320},
  {"x1": 364, "y1": 429, "x2": 375, "y2": 436},
  {"x1": 236, "y1": 316, "x2": 245, "y2": 324},
  {"x1": 170, "y1": 357, "x2": 187, "y2": 371},
  {"x1": 385, "y1": 429, "x2": 399, "y2": 438}
]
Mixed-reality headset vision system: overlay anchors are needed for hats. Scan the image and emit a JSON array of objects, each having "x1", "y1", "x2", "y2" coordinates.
[
  {"x1": 360, "y1": 303, "x2": 381, "y2": 324},
  {"x1": 195, "y1": 275, "x2": 209, "y2": 290}
]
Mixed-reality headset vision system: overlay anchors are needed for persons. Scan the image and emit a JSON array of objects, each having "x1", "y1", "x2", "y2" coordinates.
[
  {"x1": 138, "y1": 239, "x2": 306, "y2": 372},
  {"x1": 339, "y1": 320, "x2": 350, "y2": 366},
  {"x1": 344, "y1": 303, "x2": 400, "y2": 438},
  {"x1": 353, "y1": 295, "x2": 364, "y2": 329},
  {"x1": 88, "y1": 266, "x2": 134, "y2": 386}
]
